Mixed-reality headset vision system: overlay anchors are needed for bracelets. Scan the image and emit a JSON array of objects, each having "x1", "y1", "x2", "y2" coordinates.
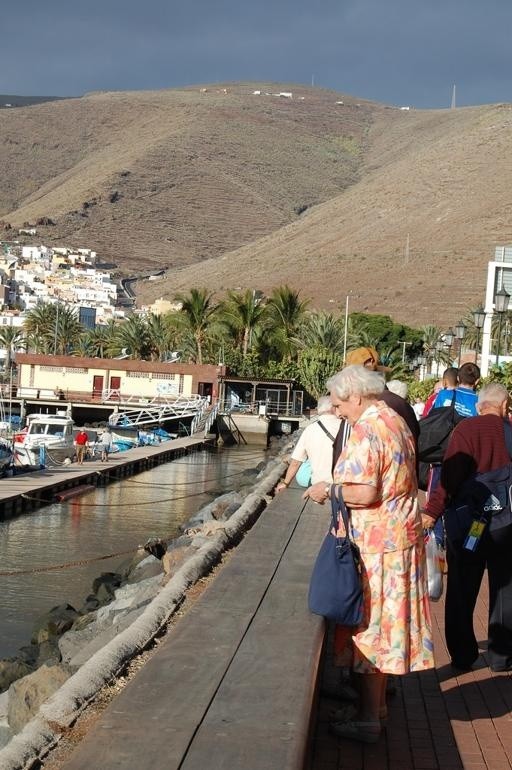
[
  {"x1": 281, "y1": 480, "x2": 289, "y2": 487},
  {"x1": 324, "y1": 482, "x2": 333, "y2": 501}
]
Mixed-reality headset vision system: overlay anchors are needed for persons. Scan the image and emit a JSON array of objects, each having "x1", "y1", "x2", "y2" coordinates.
[
  {"x1": 230, "y1": 390, "x2": 240, "y2": 409},
  {"x1": 92, "y1": 431, "x2": 100, "y2": 458},
  {"x1": 98, "y1": 427, "x2": 113, "y2": 463},
  {"x1": 418, "y1": 380, "x2": 511, "y2": 673},
  {"x1": 272, "y1": 395, "x2": 353, "y2": 495},
  {"x1": 330, "y1": 344, "x2": 422, "y2": 700},
  {"x1": 298, "y1": 364, "x2": 454, "y2": 744},
  {"x1": 74, "y1": 428, "x2": 90, "y2": 467},
  {"x1": 383, "y1": 359, "x2": 485, "y2": 553}
]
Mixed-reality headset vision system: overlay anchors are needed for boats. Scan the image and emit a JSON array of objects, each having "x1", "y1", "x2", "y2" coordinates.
[{"x1": 13, "y1": 416, "x2": 98, "y2": 470}]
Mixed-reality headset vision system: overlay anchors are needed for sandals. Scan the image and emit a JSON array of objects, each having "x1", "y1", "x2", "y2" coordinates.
[{"x1": 328, "y1": 699, "x2": 390, "y2": 742}]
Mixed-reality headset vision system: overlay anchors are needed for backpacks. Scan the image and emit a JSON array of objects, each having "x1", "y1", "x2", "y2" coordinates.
[{"x1": 415, "y1": 387, "x2": 469, "y2": 463}]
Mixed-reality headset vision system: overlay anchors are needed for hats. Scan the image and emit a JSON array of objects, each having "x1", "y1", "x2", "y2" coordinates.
[{"x1": 344, "y1": 346, "x2": 379, "y2": 371}]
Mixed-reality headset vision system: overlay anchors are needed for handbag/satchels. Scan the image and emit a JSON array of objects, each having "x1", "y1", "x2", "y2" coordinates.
[
  {"x1": 305, "y1": 482, "x2": 367, "y2": 628},
  {"x1": 440, "y1": 420, "x2": 511, "y2": 559}
]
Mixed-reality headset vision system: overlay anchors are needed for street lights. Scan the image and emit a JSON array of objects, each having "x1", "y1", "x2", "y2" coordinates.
[
  {"x1": 444, "y1": 328, "x2": 457, "y2": 368},
  {"x1": 409, "y1": 338, "x2": 444, "y2": 381},
  {"x1": 496, "y1": 284, "x2": 511, "y2": 365},
  {"x1": 455, "y1": 317, "x2": 466, "y2": 369},
  {"x1": 472, "y1": 304, "x2": 486, "y2": 365}
]
